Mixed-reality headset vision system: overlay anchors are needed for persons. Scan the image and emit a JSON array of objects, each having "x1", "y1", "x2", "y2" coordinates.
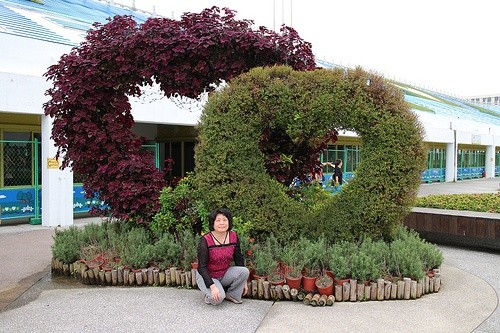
[
  {"x1": 310, "y1": 161, "x2": 333, "y2": 186},
  {"x1": 333, "y1": 159, "x2": 348, "y2": 194},
  {"x1": 195, "y1": 208, "x2": 250, "y2": 305}
]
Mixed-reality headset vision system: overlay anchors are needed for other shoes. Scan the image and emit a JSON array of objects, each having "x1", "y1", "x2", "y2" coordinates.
[{"x1": 204, "y1": 294, "x2": 243, "y2": 305}]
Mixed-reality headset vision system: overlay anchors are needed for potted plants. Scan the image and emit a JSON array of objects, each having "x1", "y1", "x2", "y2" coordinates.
[{"x1": 81, "y1": 240, "x2": 443, "y2": 296}]
